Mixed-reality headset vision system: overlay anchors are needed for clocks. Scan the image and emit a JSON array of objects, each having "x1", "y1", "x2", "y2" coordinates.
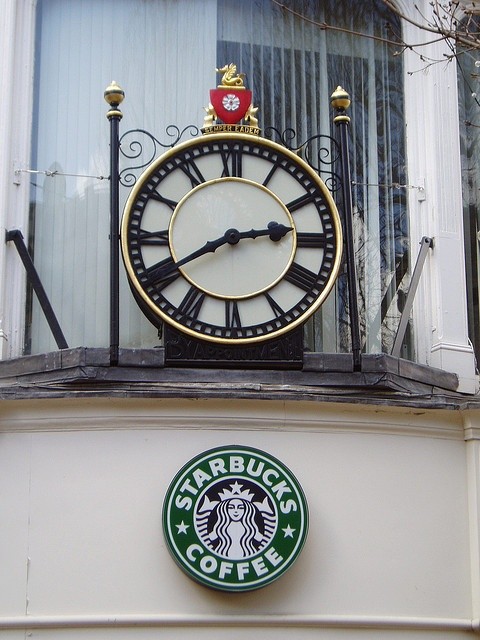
[{"x1": 120, "y1": 131, "x2": 344, "y2": 348}]
